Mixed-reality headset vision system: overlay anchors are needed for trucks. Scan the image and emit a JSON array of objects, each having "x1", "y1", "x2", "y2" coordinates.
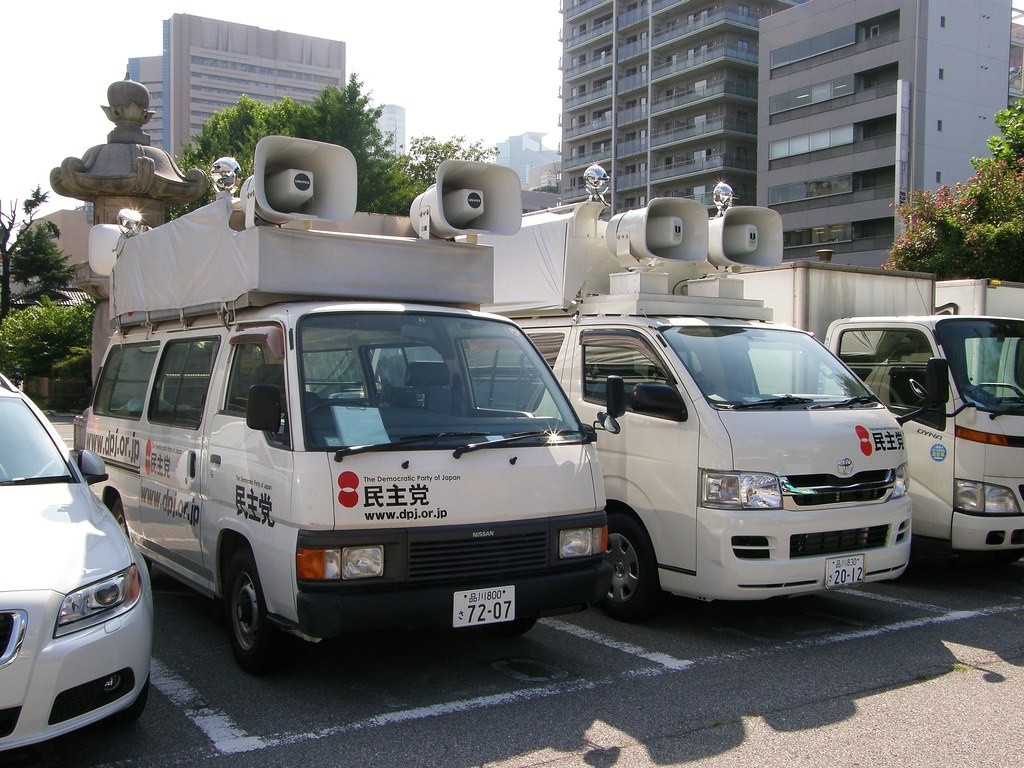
[{"x1": 738, "y1": 260, "x2": 1024, "y2": 549}]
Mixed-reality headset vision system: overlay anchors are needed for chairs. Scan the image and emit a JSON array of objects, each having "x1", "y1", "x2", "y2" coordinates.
[
  {"x1": 257, "y1": 365, "x2": 285, "y2": 413},
  {"x1": 389, "y1": 361, "x2": 455, "y2": 415}
]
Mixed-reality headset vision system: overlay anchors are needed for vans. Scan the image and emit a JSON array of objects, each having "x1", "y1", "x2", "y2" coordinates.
[
  {"x1": 72, "y1": 135, "x2": 625, "y2": 683},
  {"x1": 371, "y1": 159, "x2": 912, "y2": 624}
]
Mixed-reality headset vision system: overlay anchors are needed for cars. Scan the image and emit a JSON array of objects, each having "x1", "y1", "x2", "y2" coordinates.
[{"x1": 0, "y1": 372, "x2": 155, "y2": 761}]
